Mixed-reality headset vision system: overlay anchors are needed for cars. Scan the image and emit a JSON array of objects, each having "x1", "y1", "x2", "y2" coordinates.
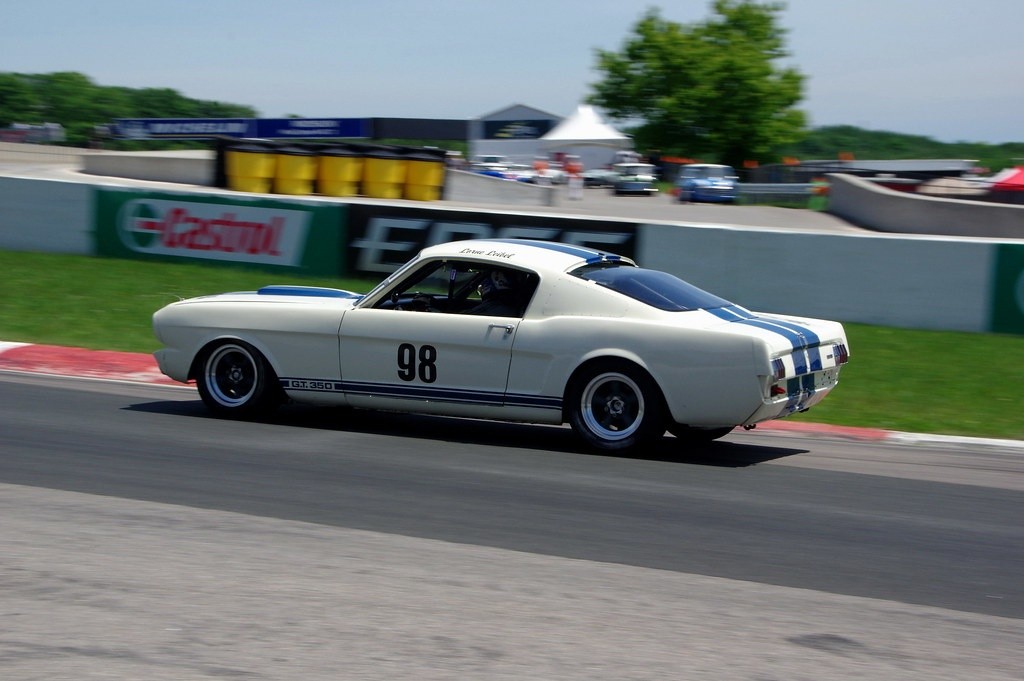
[
  {"x1": 677, "y1": 161, "x2": 739, "y2": 204},
  {"x1": 448, "y1": 168, "x2": 617, "y2": 188},
  {"x1": 609, "y1": 160, "x2": 660, "y2": 197}
]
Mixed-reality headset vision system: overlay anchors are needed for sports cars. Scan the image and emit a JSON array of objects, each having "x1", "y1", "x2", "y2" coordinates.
[{"x1": 151, "y1": 237, "x2": 852, "y2": 455}]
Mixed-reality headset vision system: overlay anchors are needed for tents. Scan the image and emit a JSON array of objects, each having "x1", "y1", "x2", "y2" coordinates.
[{"x1": 537, "y1": 103, "x2": 635, "y2": 156}]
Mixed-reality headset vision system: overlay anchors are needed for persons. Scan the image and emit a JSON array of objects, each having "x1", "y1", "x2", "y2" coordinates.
[
  {"x1": 398, "y1": 269, "x2": 519, "y2": 317},
  {"x1": 564, "y1": 155, "x2": 584, "y2": 201},
  {"x1": 532, "y1": 156, "x2": 550, "y2": 175}
]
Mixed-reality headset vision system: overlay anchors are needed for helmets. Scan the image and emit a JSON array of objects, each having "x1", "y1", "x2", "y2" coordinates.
[{"x1": 480, "y1": 270, "x2": 519, "y2": 297}]
